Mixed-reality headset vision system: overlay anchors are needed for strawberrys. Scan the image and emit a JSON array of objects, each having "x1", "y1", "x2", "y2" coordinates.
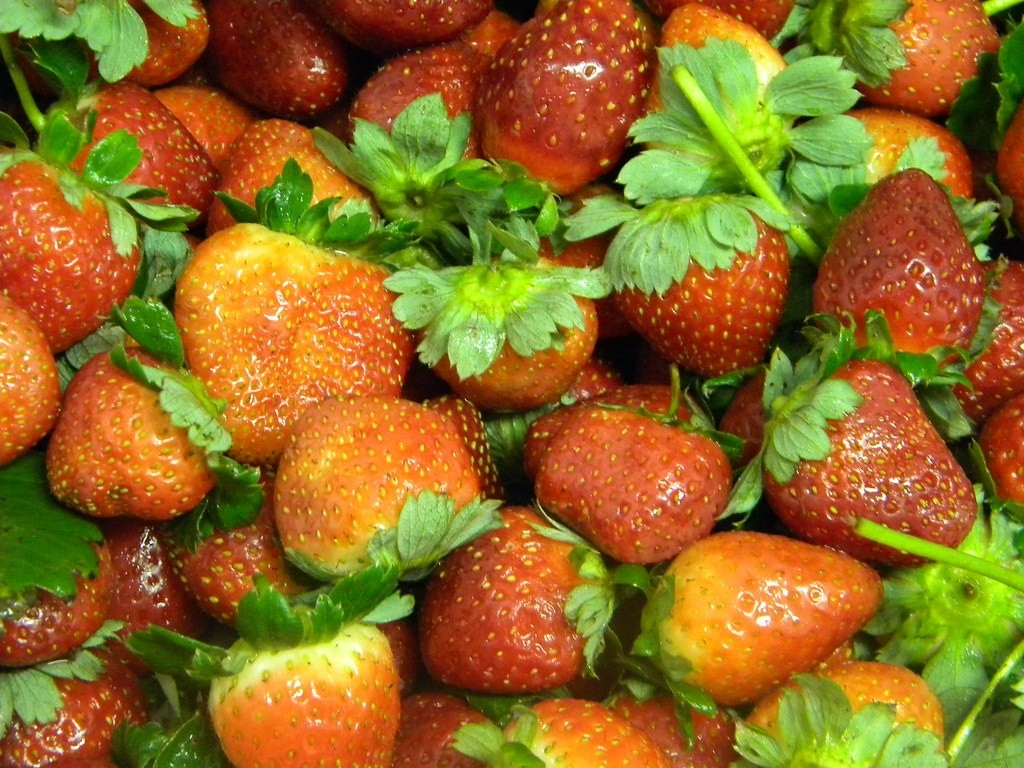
[{"x1": 0, "y1": 2, "x2": 1024, "y2": 766}]
